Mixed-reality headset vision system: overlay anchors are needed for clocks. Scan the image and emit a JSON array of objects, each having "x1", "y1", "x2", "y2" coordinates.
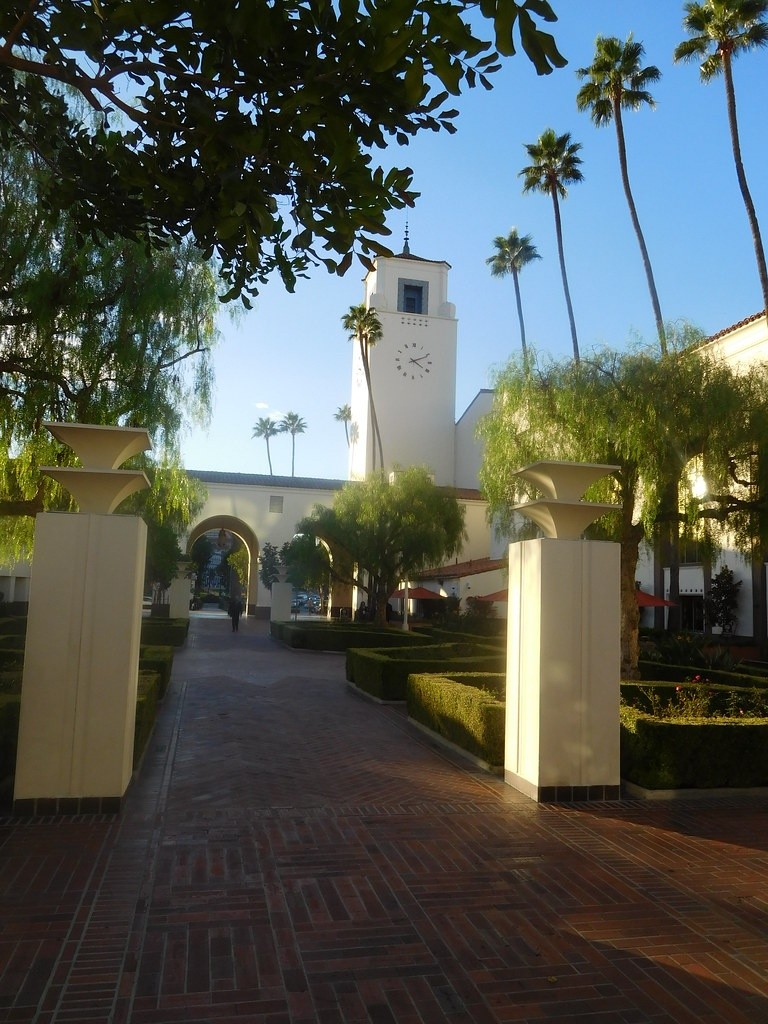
[{"x1": 395, "y1": 343, "x2": 433, "y2": 380}]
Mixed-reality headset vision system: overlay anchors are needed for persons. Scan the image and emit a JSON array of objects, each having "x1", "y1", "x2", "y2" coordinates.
[
  {"x1": 386, "y1": 601, "x2": 392, "y2": 624},
  {"x1": 228, "y1": 595, "x2": 244, "y2": 632},
  {"x1": 307, "y1": 597, "x2": 314, "y2": 616},
  {"x1": 193, "y1": 596, "x2": 199, "y2": 610},
  {"x1": 358, "y1": 601, "x2": 377, "y2": 623}
]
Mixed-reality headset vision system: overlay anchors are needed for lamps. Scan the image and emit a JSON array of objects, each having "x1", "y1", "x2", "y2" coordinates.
[{"x1": 217, "y1": 527, "x2": 227, "y2": 547}]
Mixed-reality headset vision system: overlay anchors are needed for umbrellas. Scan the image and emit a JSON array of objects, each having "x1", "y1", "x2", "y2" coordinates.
[
  {"x1": 478, "y1": 587, "x2": 507, "y2": 602},
  {"x1": 634, "y1": 590, "x2": 678, "y2": 608},
  {"x1": 391, "y1": 586, "x2": 447, "y2": 616}
]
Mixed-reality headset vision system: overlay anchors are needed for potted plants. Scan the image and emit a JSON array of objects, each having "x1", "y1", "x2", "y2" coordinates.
[{"x1": 701, "y1": 565, "x2": 743, "y2": 638}]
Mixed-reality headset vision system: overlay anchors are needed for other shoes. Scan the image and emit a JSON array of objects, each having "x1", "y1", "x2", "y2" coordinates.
[{"x1": 232, "y1": 630, "x2": 235, "y2": 632}]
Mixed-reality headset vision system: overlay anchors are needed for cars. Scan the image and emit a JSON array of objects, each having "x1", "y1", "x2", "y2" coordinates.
[{"x1": 291, "y1": 594, "x2": 321, "y2": 612}]
